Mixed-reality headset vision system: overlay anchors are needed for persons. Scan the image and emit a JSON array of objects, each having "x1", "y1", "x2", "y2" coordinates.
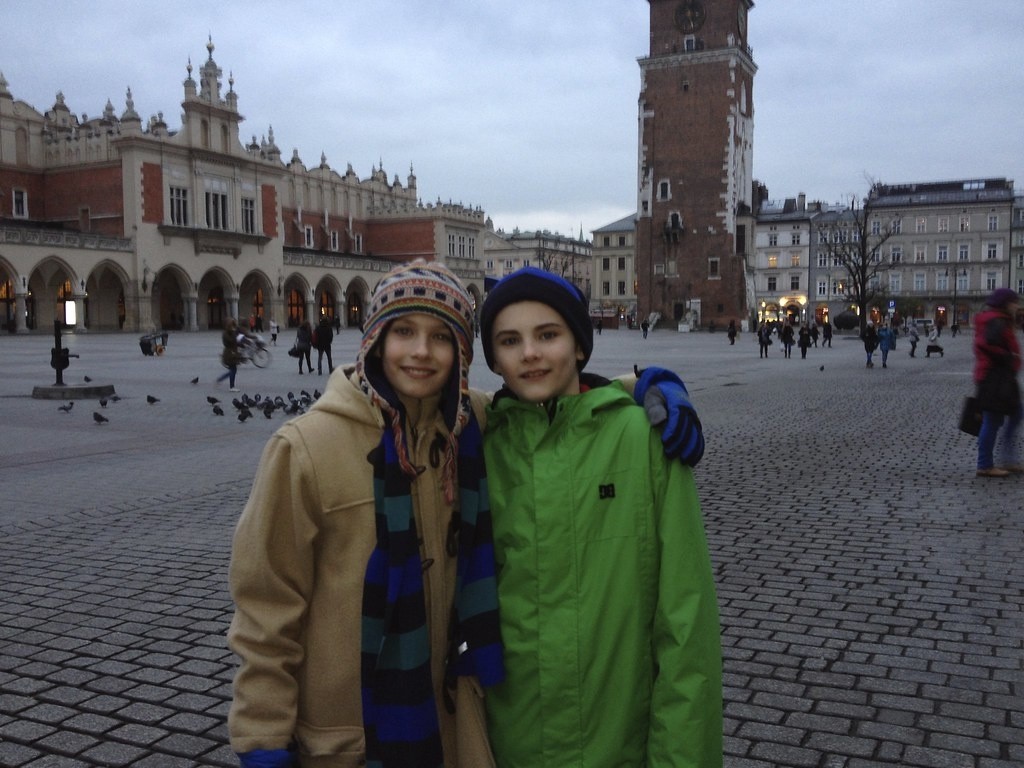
[
  {"x1": 759, "y1": 318, "x2": 794, "y2": 359},
  {"x1": 596, "y1": 320, "x2": 602, "y2": 335},
  {"x1": 642, "y1": 320, "x2": 649, "y2": 339},
  {"x1": 314, "y1": 315, "x2": 335, "y2": 375},
  {"x1": 479, "y1": 266, "x2": 723, "y2": 768},
  {"x1": 959, "y1": 286, "x2": 1024, "y2": 477},
  {"x1": 800, "y1": 321, "x2": 820, "y2": 359},
  {"x1": 249, "y1": 313, "x2": 263, "y2": 332},
  {"x1": 822, "y1": 319, "x2": 832, "y2": 347},
  {"x1": 236, "y1": 326, "x2": 257, "y2": 338},
  {"x1": 335, "y1": 315, "x2": 341, "y2": 335},
  {"x1": 860, "y1": 320, "x2": 899, "y2": 369},
  {"x1": 297, "y1": 321, "x2": 315, "y2": 375},
  {"x1": 227, "y1": 258, "x2": 706, "y2": 768},
  {"x1": 909, "y1": 322, "x2": 920, "y2": 357},
  {"x1": 727, "y1": 319, "x2": 735, "y2": 346},
  {"x1": 924, "y1": 322, "x2": 962, "y2": 338},
  {"x1": 214, "y1": 318, "x2": 241, "y2": 391},
  {"x1": 269, "y1": 319, "x2": 279, "y2": 347},
  {"x1": 925, "y1": 325, "x2": 944, "y2": 358}
]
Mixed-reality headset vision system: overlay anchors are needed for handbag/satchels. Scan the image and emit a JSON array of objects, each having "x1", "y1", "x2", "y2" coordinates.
[
  {"x1": 781, "y1": 342, "x2": 785, "y2": 352},
  {"x1": 791, "y1": 339, "x2": 796, "y2": 346},
  {"x1": 958, "y1": 396, "x2": 1004, "y2": 437},
  {"x1": 768, "y1": 340, "x2": 773, "y2": 345},
  {"x1": 288, "y1": 346, "x2": 301, "y2": 359},
  {"x1": 277, "y1": 325, "x2": 279, "y2": 333}
]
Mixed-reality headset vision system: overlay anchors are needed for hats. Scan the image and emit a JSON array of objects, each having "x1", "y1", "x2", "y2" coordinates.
[
  {"x1": 867, "y1": 320, "x2": 874, "y2": 325},
  {"x1": 356, "y1": 257, "x2": 477, "y2": 504},
  {"x1": 986, "y1": 288, "x2": 1019, "y2": 307},
  {"x1": 481, "y1": 268, "x2": 593, "y2": 376}
]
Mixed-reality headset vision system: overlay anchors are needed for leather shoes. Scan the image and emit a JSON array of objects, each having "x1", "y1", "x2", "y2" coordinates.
[{"x1": 976, "y1": 467, "x2": 1010, "y2": 477}]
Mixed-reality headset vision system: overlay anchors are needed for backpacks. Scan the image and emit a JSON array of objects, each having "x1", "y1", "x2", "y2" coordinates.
[{"x1": 311, "y1": 327, "x2": 319, "y2": 349}]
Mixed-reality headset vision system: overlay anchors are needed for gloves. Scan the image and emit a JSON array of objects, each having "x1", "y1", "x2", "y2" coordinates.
[
  {"x1": 635, "y1": 366, "x2": 706, "y2": 469},
  {"x1": 237, "y1": 749, "x2": 299, "y2": 768}
]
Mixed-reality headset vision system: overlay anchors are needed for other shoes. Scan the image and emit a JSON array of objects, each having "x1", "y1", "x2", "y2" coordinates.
[
  {"x1": 228, "y1": 387, "x2": 240, "y2": 392},
  {"x1": 866, "y1": 362, "x2": 874, "y2": 368},
  {"x1": 925, "y1": 355, "x2": 930, "y2": 358},
  {"x1": 882, "y1": 363, "x2": 888, "y2": 369},
  {"x1": 940, "y1": 351, "x2": 944, "y2": 357},
  {"x1": 309, "y1": 368, "x2": 315, "y2": 373},
  {"x1": 330, "y1": 367, "x2": 335, "y2": 374}
]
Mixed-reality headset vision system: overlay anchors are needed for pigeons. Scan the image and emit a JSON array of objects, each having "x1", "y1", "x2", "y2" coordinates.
[
  {"x1": 206, "y1": 397, "x2": 219, "y2": 405},
  {"x1": 213, "y1": 406, "x2": 224, "y2": 416},
  {"x1": 191, "y1": 377, "x2": 198, "y2": 384},
  {"x1": 232, "y1": 388, "x2": 314, "y2": 421},
  {"x1": 84, "y1": 376, "x2": 92, "y2": 383},
  {"x1": 111, "y1": 395, "x2": 121, "y2": 402},
  {"x1": 99, "y1": 397, "x2": 108, "y2": 407},
  {"x1": 147, "y1": 395, "x2": 160, "y2": 404},
  {"x1": 820, "y1": 365, "x2": 824, "y2": 371},
  {"x1": 313, "y1": 389, "x2": 321, "y2": 400},
  {"x1": 93, "y1": 412, "x2": 109, "y2": 424},
  {"x1": 58, "y1": 402, "x2": 74, "y2": 413}
]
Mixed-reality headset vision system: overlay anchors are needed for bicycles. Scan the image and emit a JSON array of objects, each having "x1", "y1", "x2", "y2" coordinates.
[{"x1": 221, "y1": 343, "x2": 271, "y2": 369}]
